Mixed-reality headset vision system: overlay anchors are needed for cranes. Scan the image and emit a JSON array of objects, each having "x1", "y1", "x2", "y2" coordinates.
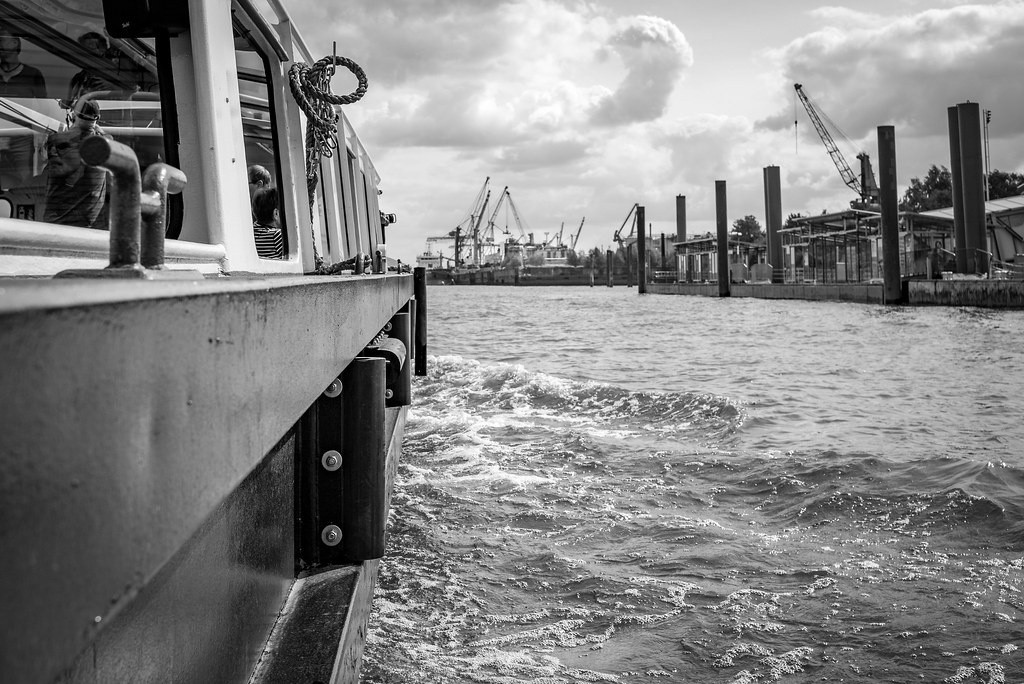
[
  {"x1": 417, "y1": 176, "x2": 586, "y2": 278},
  {"x1": 794, "y1": 82, "x2": 881, "y2": 221}
]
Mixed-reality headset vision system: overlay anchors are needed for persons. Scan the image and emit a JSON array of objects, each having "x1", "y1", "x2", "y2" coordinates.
[
  {"x1": 0, "y1": 30, "x2": 162, "y2": 99},
  {"x1": 43, "y1": 99, "x2": 113, "y2": 230},
  {"x1": 931, "y1": 241, "x2": 943, "y2": 279},
  {"x1": 247, "y1": 165, "x2": 285, "y2": 259}
]
